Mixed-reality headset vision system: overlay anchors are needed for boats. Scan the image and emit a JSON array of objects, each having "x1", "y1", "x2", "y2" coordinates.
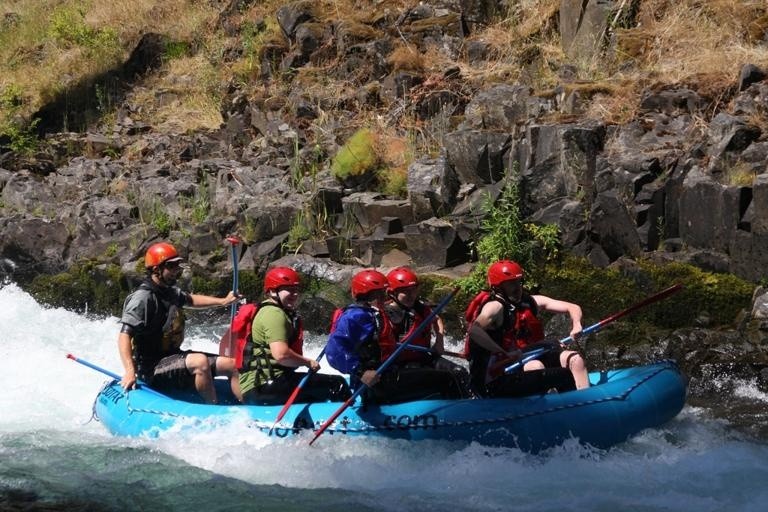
[{"x1": 93, "y1": 351, "x2": 690, "y2": 454}]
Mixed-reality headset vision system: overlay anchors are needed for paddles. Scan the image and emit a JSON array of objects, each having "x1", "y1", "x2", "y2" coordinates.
[
  {"x1": 267, "y1": 309, "x2": 342, "y2": 436},
  {"x1": 218, "y1": 237, "x2": 240, "y2": 356},
  {"x1": 503, "y1": 282, "x2": 683, "y2": 377},
  {"x1": 309, "y1": 285, "x2": 462, "y2": 445}
]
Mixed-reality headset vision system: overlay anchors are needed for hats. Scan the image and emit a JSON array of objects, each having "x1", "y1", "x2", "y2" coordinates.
[{"x1": 166, "y1": 257, "x2": 185, "y2": 262}]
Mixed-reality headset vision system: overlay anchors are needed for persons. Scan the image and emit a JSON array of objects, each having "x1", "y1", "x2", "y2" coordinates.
[
  {"x1": 238, "y1": 264, "x2": 355, "y2": 404},
  {"x1": 117, "y1": 241, "x2": 242, "y2": 406},
  {"x1": 459, "y1": 258, "x2": 590, "y2": 396},
  {"x1": 324, "y1": 270, "x2": 467, "y2": 406},
  {"x1": 384, "y1": 266, "x2": 467, "y2": 389}
]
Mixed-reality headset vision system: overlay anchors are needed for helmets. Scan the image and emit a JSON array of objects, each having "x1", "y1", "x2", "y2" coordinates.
[
  {"x1": 386, "y1": 268, "x2": 418, "y2": 292},
  {"x1": 144, "y1": 243, "x2": 177, "y2": 270},
  {"x1": 264, "y1": 267, "x2": 300, "y2": 293},
  {"x1": 489, "y1": 261, "x2": 523, "y2": 287},
  {"x1": 353, "y1": 270, "x2": 390, "y2": 298}
]
